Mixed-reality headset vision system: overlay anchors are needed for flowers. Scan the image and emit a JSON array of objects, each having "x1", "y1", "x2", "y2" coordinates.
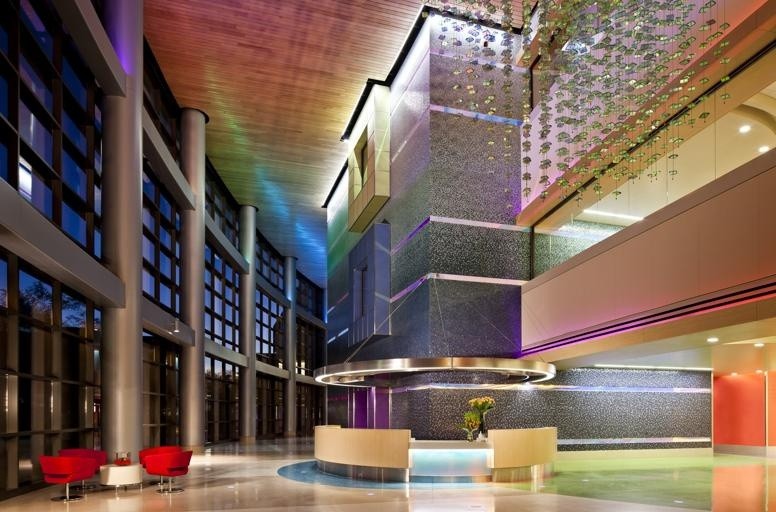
[{"x1": 460, "y1": 395, "x2": 495, "y2": 441}]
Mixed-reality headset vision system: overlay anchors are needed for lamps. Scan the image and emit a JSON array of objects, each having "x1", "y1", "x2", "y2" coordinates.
[{"x1": 312, "y1": 267, "x2": 560, "y2": 396}]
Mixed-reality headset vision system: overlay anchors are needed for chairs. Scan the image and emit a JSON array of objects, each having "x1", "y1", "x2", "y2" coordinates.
[
  {"x1": 38, "y1": 442, "x2": 106, "y2": 502},
  {"x1": 136, "y1": 443, "x2": 194, "y2": 495}
]
